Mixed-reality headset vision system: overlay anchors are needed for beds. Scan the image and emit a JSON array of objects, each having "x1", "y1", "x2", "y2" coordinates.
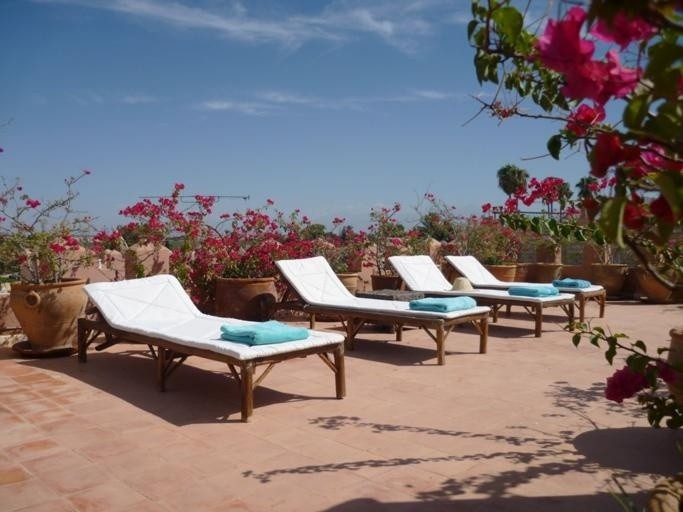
[
  {"x1": 444, "y1": 253, "x2": 606, "y2": 325},
  {"x1": 82, "y1": 274, "x2": 346, "y2": 423},
  {"x1": 388, "y1": 254, "x2": 575, "y2": 337},
  {"x1": 275, "y1": 255, "x2": 491, "y2": 365}
]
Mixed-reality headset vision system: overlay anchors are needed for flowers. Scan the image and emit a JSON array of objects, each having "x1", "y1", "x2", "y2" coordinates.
[
  {"x1": 452, "y1": 206, "x2": 456, "y2": 209},
  {"x1": 495, "y1": 101, "x2": 510, "y2": 117},
  {"x1": 568, "y1": 102, "x2": 682, "y2": 234},
  {"x1": 564, "y1": 320, "x2": 682, "y2": 432},
  {"x1": 314, "y1": 193, "x2": 466, "y2": 270},
  {"x1": 471, "y1": 177, "x2": 571, "y2": 263},
  {"x1": 527, "y1": 7, "x2": 659, "y2": 105},
  {"x1": 65, "y1": 179, "x2": 67, "y2": 182},
  {"x1": 1, "y1": 171, "x2": 313, "y2": 303}
]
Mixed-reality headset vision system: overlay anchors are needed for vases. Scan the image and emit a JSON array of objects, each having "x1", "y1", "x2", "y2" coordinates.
[
  {"x1": 370, "y1": 275, "x2": 403, "y2": 291},
  {"x1": 667, "y1": 327, "x2": 682, "y2": 410},
  {"x1": 9, "y1": 277, "x2": 88, "y2": 354},
  {"x1": 336, "y1": 274, "x2": 359, "y2": 296},
  {"x1": 487, "y1": 263, "x2": 586, "y2": 283},
  {"x1": 215, "y1": 277, "x2": 280, "y2": 322},
  {"x1": 588, "y1": 262, "x2": 682, "y2": 298}
]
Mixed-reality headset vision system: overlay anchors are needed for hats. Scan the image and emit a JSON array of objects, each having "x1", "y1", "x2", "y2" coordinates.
[{"x1": 444, "y1": 277, "x2": 480, "y2": 293}]
